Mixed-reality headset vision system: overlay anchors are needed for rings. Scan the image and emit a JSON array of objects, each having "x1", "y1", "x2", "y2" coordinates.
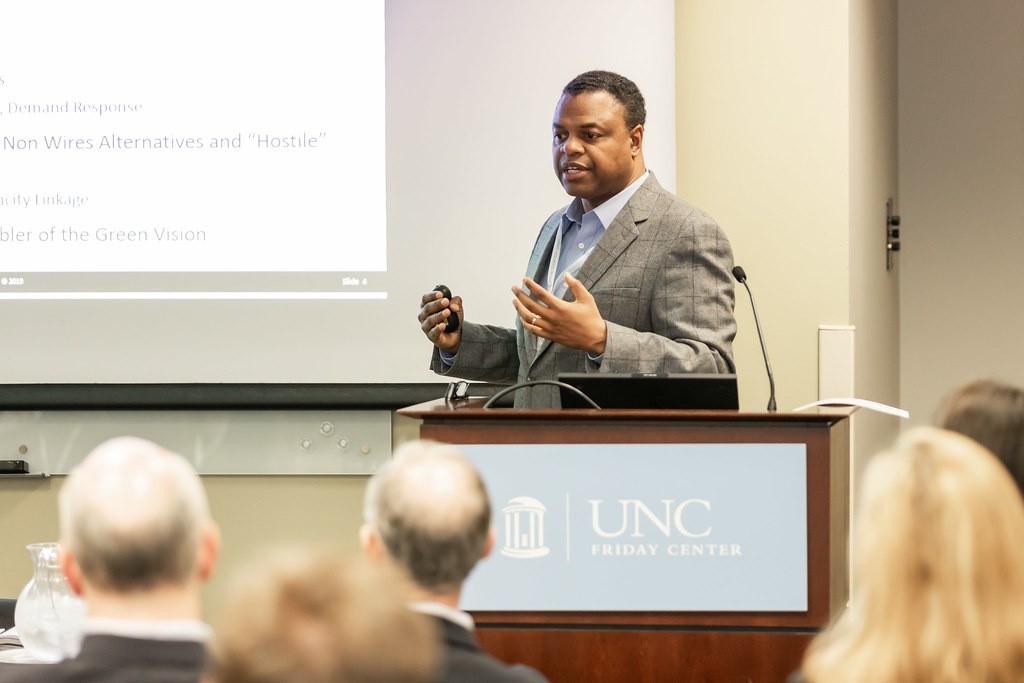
[{"x1": 532, "y1": 315, "x2": 537, "y2": 325}]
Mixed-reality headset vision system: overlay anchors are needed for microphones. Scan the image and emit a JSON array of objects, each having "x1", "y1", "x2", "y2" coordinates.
[{"x1": 732, "y1": 265, "x2": 777, "y2": 411}]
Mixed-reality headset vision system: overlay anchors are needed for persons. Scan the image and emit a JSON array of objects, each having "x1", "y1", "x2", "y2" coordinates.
[
  {"x1": 356, "y1": 441, "x2": 550, "y2": 683},
  {"x1": 419, "y1": 70, "x2": 733, "y2": 410},
  {"x1": 785, "y1": 427, "x2": 1024, "y2": 682},
  {"x1": 938, "y1": 382, "x2": 1024, "y2": 504},
  {"x1": 0, "y1": 436, "x2": 222, "y2": 681},
  {"x1": 197, "y1": 542, "x2": 441, "y2": 683}
]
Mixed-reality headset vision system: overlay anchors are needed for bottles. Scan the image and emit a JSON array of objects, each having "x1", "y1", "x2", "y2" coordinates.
[{"x1": 14, "y1": 543, "x2": 86, "y2": 667}]
